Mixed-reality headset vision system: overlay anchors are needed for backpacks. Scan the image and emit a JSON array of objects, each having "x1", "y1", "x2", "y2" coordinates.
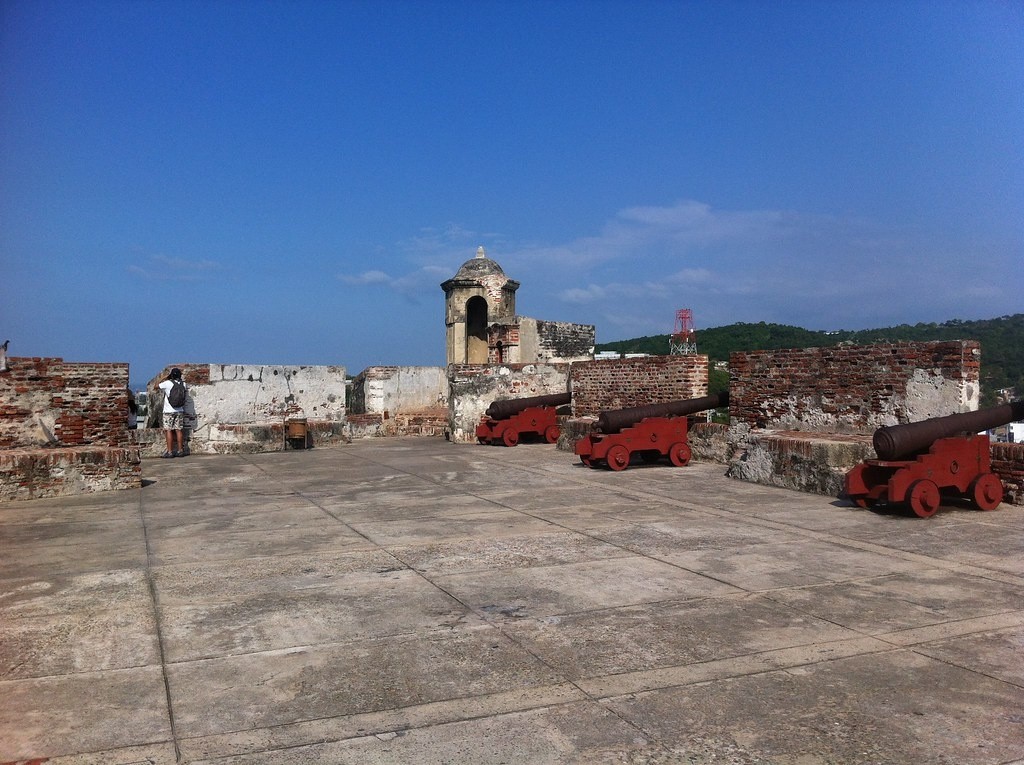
[{"x1": 165, "y1": 379, "x2": 185, "y2": 407}]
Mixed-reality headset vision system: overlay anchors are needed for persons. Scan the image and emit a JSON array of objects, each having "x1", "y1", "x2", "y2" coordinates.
[
  {"x1": 127, "y1": 390, "x2": 138, "y2": 430},
  {"x1": 154, "y1": 368, "x2": 186, "y2": 458}
]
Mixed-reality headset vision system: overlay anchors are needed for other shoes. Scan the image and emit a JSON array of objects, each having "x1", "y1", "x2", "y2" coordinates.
[
  {"x1": 163, "y1": 452, "x2": 173, "y2": 457},
  {"x1": 174, "y1": 452, "x2": 184, "y2": 457}
]
